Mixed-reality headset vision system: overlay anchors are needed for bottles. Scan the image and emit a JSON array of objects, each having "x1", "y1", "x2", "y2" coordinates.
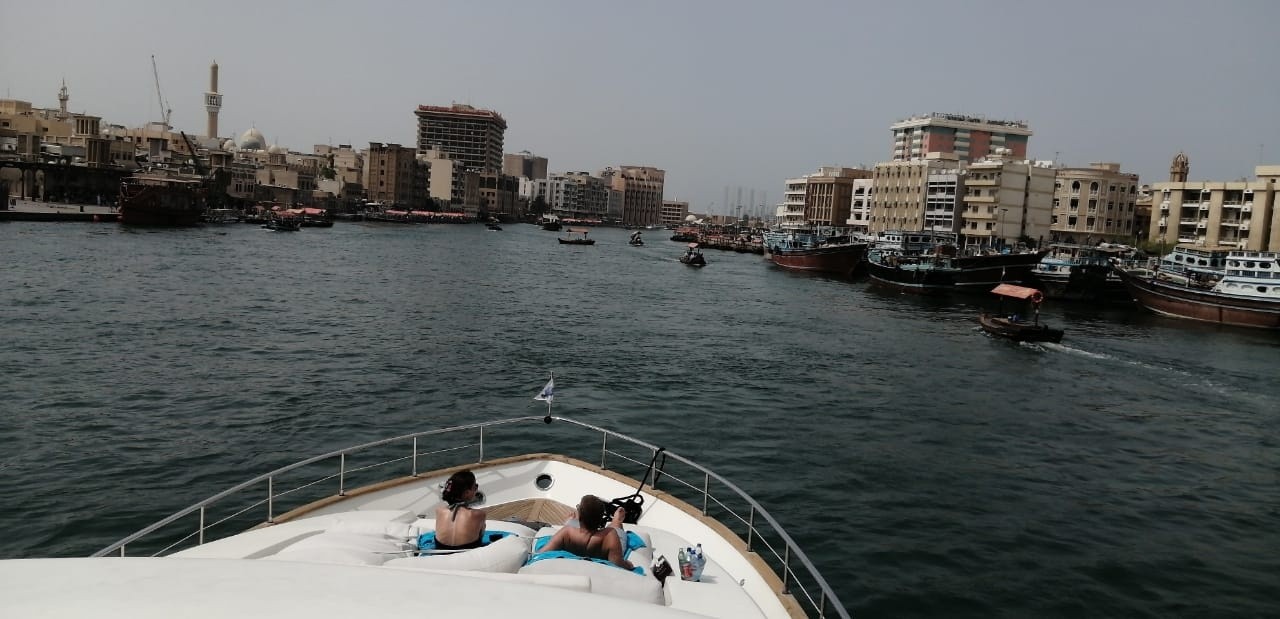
[{"x1": 678, "y1": 543, "x2": 703, "y2": 578}]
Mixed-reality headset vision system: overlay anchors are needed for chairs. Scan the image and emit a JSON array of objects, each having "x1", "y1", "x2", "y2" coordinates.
[
  {"x1": 385, "y1": 519, "x2": 533, "y2": 576},
  {"x1": 519, "y1": 523, "x2": 665, "y2": 604}
]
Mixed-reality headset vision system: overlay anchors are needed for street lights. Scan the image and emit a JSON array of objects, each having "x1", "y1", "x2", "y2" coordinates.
[{"x1": 1000, "y1": 208, "x2": 1009, "y2": 253}]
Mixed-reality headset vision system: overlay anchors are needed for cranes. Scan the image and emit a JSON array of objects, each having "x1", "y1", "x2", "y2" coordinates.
[{"x1": 151, "y1": 55, "x2": 172, "y2": 126}]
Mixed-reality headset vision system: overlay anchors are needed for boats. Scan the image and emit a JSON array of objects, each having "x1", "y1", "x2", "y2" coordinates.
[
  {"x1": 202, "y1": 208, "x2": 241, "y2": 225},
  {"x1": 245, "y1": 206, "x2": 334, "y2": 232},
  {"x1": 1111, "y1": 245, "x2": 1280, "y2": 331},
  {"x1": 544, "y1": 222, "x2": 563, "y2": 231},
  {"x1": 979, "y1": 282, "x2": 1064, "y2": 345},
  {"x1": 630, "y1": 231, "x2": 644, "y2": 246},
  {"x1": 770, "y1": 222, "x2": 1138, "y2": 306},
  {"x1": 485, "y1": 216, "x2": 502, "y2": 231},
  {"x1": 680, "y1": 243, "x2": 705, "y2": 264},
  {"x1": 558, "y1": 227, "x2": 596, "y2": 245},
  {"x1": 116, "y1": 176, "x2": 204, "y2": 228}
]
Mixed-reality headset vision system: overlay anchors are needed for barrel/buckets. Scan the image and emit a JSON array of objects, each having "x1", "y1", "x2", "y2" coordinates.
[{"x1": 679, "y1": 557, "x2": 707, "y2": 582}]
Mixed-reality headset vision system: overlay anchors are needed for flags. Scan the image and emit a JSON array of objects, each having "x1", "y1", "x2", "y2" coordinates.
[{"x1": 533, "y1": 378, "x2": 554, "y2": 404}]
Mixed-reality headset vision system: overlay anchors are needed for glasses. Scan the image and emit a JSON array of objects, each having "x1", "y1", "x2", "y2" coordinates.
[
  {"x1": 576, "y1": 504, "x2": 580, "y2": 512},
  {"x1": 472, "y1": 484, "x2": 478, "y2": 491}
]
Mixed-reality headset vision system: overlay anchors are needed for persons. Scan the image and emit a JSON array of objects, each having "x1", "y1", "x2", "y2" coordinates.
[
  {"x1": 434, "y1": 470, "x2": 486, "y2": 552},
  {"x1": 536, "y1": 494, "x2": 636, "y2": 570}
]
